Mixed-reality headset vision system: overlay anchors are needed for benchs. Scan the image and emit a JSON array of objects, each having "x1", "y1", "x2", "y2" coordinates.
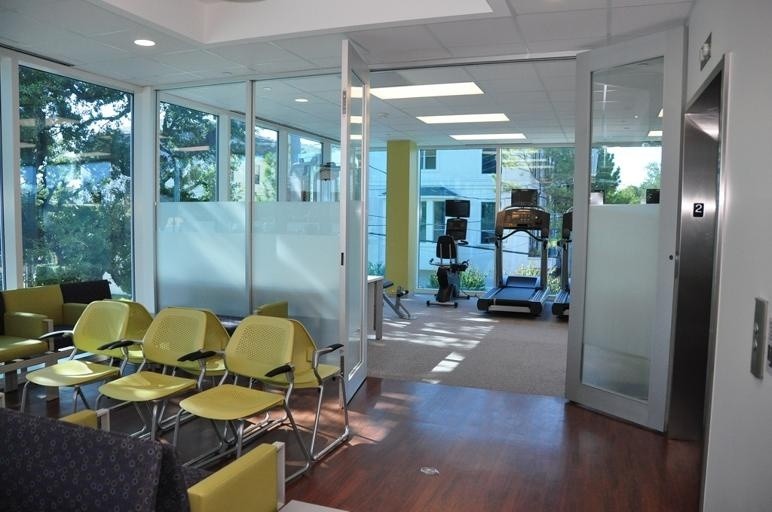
[{"x1": 0, "y1": 395, "x2": 290, "y2": 512}]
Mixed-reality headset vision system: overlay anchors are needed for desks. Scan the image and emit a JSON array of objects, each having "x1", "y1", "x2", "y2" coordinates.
[{"x1": 367, "y1": 275, "x2": 384, "y2": 340}]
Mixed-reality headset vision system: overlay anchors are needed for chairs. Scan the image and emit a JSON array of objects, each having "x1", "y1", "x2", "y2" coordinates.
[
  {"x1": 0, "y1": 281, "x2": 152, "y2": 421},
  {"x1": 261, "y1": 319, "x2": 351, "y2": 461},
  {"x1": 255, "y1": 301, "x2": 291, "y2": 318},
  {"x1": 170, "y1": 307, "x2": 242, "y2": 445},
  {"x1": 96, "y1": 306, "x2": 209, "y2": 468},
  {"x1": 173, "y1": 315, "x2": 310, "y2": 482}
]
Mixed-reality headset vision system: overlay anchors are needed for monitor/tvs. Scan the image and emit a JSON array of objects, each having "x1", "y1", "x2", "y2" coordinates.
[
  {"x1": 445, "y1": 218, "x2": 467, "y2": 241},
  {"x1": 590, "y1": 190, "x2": 606, "y2": 205},
  {"x1": 646, "y1": 189, "x2": 660, "y2": 205},
  {"x1": 511, "y1": 188, "x2": 539, "y2": 208},
  {"x1": 444, "y1": 199, "x2": 471, "y2": 218}
]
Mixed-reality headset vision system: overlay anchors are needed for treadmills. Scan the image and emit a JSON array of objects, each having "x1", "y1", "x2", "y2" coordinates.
[
  {"x1": 476, "y1": 189, "x2": 550, "y2": 313},
  {"x1": 552, "y1": 207, "x2": 573, "y2": 315}
]
